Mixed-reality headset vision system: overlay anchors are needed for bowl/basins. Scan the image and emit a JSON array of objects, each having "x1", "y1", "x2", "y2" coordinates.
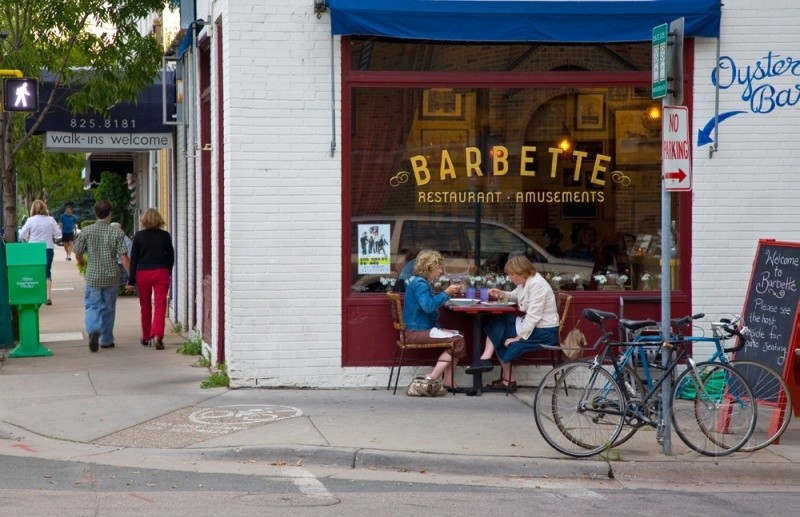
[
  {"x1": 449, "y1": 298, "x2": 474, "y2": 308},
  {"x1": 472, "y1": 299, "x2": 481, "y2": 305}
]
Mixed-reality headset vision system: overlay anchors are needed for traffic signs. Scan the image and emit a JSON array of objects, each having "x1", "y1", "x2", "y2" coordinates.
[{"x1": 661, "y1": 107, "x2": 692, "y2": 192}]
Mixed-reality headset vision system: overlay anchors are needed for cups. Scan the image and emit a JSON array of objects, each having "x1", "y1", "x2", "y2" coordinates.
[
  {"x1": 479, "y1": 286, "x2": 489, "y2": 302},
  {"x1": 466, "y1": 288, "x2": 475, "y2": 299}
]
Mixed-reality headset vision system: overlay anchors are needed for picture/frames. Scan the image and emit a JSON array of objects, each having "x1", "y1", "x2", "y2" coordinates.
[
  {"x1": 418, "y1": 88, "x2": 466, "y2": 122},
  {"x1": 525, "y1": 92, "x2": 606, "y2": 219},
  {"x1": 420, "y1": 128, "x2": 470, "y2": 166}
]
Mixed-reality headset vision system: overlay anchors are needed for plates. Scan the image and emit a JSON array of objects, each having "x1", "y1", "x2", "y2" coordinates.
[{"x1": 480, "y1": 300, "x2": 516, "y2": 307}]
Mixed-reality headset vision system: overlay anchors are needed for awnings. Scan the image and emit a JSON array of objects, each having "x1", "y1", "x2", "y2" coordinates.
[{"x1": 314, "y1": 0, "x2": 721, "y2": 43}]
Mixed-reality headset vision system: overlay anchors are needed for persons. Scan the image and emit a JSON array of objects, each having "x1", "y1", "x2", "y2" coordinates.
[
  {"x1": 19, "y1": 199, "x2": 63, "y2": 306},
  {"x1": 540, "y1": 225, "x2": 566, "y2": 258},
  {"x1": 360, "y1": 231, "x2": 388, "y2": 257},
  {"x1": 464, "y1": 255, "x2": 559, "y2": 392},
  {"x1": 399, "y1": 250, "x2": 464, "y2": 396},
  {"x1": 110, "y1": 222, "x2": 134, "y2": 283},
  {"x1": 74, "y1": 200, "x2": 130, "y2": 352},
  {"x1": 127, "y1": 208, "x2": 175, "y2": 351},
  {"x1": 58, "y1": 205, "x2": 77, "y2": 261}
]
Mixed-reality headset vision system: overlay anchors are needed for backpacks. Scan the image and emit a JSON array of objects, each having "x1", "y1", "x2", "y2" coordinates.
[{"x1": 559, "y1": 297, "x2": 588, "y2": 359}]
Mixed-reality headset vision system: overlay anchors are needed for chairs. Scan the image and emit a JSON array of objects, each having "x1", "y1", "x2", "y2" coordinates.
[
  {"x1": 501, "y1": 293, "x2": 572, "y2": 396},
  {"x1": 386, "y1": 290, "x2": 455, "y2": 395}
]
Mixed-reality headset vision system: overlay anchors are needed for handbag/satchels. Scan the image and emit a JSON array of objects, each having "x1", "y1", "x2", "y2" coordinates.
[{"x1": 407, "y1": 376, "x2": 446, "y2": 398}]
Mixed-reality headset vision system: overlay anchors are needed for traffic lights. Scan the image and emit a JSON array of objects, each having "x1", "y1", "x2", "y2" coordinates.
[{"x1": 0, "y1": 76, "x2": 41, "y2": 113}]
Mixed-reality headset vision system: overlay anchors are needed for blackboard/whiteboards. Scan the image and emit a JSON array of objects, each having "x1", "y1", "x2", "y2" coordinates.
[{"x1": 725, "y1": 240, "x2": 800, "y2": 406}]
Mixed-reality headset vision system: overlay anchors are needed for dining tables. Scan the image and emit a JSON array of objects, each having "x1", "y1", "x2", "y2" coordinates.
[{"x1": 444, "y1": 301, "x2": 516, "y2": 395}]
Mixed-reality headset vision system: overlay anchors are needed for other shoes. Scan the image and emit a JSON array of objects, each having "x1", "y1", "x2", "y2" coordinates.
[
  {"x1": 154, "y1": 336, "x2": 164, "y2": 349},
  {"x1": 101, "y1": 343, "x2": 115, "y2": 348},
  {"x1": 46, "y1": 299, "x2": 52, "y2": 305},
  {"x1": 89, "y1": 328, "x2": 100, "y2": 352},
  {"x1": 140, "y1": 340, "x2": 151, "y2": 345}
]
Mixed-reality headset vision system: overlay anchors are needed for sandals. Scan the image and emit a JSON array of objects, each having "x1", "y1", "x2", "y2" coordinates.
[
  {"x1": 466, "y1": 359, "x2": 494, "y2": 374},
  {"x1": 486, "y1": 378, "x2": 517, "y2": 393}
]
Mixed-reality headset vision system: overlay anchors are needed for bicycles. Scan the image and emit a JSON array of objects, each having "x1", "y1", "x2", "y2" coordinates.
[
  {"x1": 552, "y1": 311, "x2": 792, "y2": 452},
  {"x1": 534, "y1": 306, "x2": 758, "y2": 458}
]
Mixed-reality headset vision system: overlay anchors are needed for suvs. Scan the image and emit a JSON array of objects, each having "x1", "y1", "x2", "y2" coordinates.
[{"x1": 350, "y1": 213, "x2": 620, "y2": 293}]
